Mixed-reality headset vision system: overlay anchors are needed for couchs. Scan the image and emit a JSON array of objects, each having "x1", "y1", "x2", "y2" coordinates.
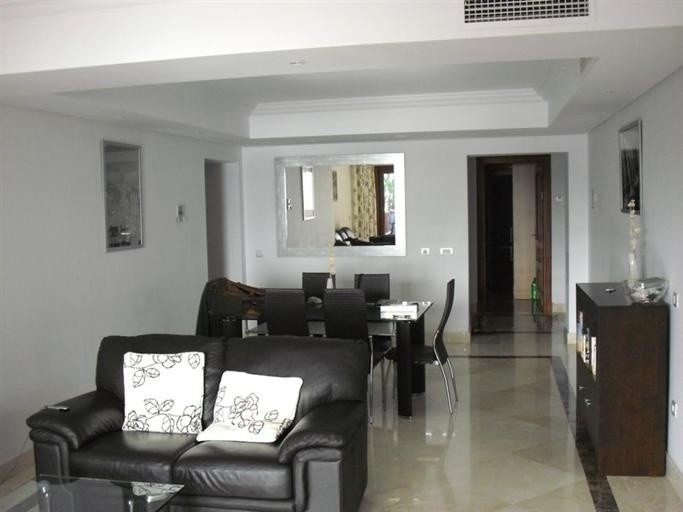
[
  {"x1": 26, "y1": 335, "x2": 370, "y2": 512},
  {"x1": 335, "y1": 227, "x2": 354, "y2": 246}
]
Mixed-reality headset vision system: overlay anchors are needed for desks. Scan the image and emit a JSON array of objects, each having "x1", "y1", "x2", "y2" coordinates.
[{"x1": 240, "y1": 291, "x2": 434, "y2": 420}]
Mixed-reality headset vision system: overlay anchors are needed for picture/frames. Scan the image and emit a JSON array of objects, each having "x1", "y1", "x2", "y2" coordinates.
[
  {"x1": 301, "y1": 166, "x2": 315, "y2": 221},
  {"x1": 619, "y1": 119, "x2": 643, "y2": 216},
  {"x1": 100, "y1": 139, "x2": 144, "y2": 252}
]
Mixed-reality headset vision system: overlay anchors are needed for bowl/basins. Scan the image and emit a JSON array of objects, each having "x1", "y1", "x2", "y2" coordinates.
[{"x1": 624, "y1": 279, "x2": 668, "y2": 306}]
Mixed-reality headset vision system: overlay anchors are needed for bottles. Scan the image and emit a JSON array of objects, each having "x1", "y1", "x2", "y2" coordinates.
[
  {"x1": 530, "y1": 277, "x2": 537, "y2": 300},
  {"x1": 531, "y1": 300, "x2": 535, "y2": 321}
]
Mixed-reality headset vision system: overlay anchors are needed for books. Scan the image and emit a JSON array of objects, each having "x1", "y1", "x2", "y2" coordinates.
[{"x1": 377, "y1": 299, "x2": 426, "y2": 312}]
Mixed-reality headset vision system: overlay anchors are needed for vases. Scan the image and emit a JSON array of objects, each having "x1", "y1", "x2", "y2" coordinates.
[{"x1": 627, "y1": 252, "x2": 642, "y2": 289}]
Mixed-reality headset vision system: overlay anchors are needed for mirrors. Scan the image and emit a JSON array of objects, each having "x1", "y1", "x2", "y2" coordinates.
[{"x1": 274, "y1": 152, "x2": 406, "y2": 257}]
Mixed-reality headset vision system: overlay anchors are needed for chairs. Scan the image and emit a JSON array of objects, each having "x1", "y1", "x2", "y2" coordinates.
[
  {"x1": 301, "y1": 273, "x2": 395, "y2": 400},
  {"x1": 263, "y1": 288, "x2": 387, "y2": 425},
  {"x1": 381, "y1": 279, "x2": 458, "y2": 417}
]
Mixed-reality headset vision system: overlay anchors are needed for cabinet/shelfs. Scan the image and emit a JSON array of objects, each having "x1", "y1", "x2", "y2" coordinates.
[{"x1": 577, "y1": 282, "x2": 670, "y2": 477}]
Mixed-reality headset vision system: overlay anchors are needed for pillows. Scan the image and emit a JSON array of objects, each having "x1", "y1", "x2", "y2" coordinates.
[
  {"x1": 196, "y1": 371, "x2": 303, "y2": 443},
  {"x1": 121, "y1": 351, "x2": 205, "y2": 435}
]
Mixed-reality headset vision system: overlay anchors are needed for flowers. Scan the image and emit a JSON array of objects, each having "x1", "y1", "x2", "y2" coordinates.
[{"x1": 627, "y1": 200, "x2": 642, "y2": 279}]
